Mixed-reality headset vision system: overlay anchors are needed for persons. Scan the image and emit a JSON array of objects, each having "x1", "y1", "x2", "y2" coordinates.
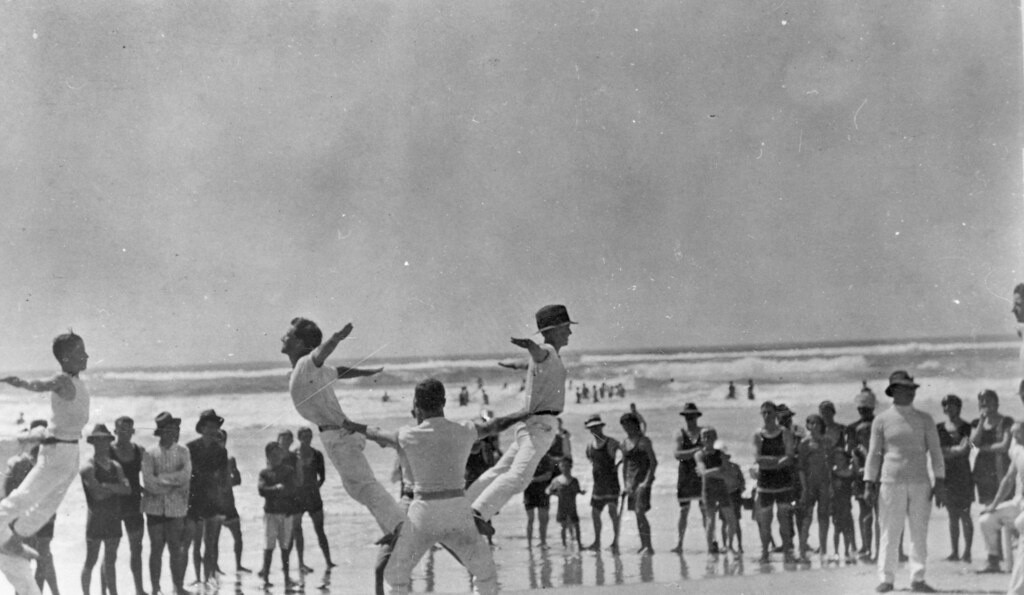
[
  {"x1": 0, "y1": 407, "x2": 338, "y2": 595},
  {"x1": 862, "y1": 369, "x2": 946, "y2": 593},
  {"x1": 275, "y1": 317, "x2": 404, "y2": 543},
  {"x1": 0, "y1": 329, "x2": 91, "y2": 539},
  {"x1": 582, "y1": 282, "x2": 1024, "y2": 594},
  {"x1": 341, "y1": 375, "x2": 533, "y2": 595},
  {"x1": 464, "y1": 304, "x2": 579, "y2": 536},
  {"x1": 364, "y1": 374, "x2": 585, "y2": 595}
]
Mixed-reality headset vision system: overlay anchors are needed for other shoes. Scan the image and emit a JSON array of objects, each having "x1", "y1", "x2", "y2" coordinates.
[
  {"x1": 913, "y1": 581, "x2": 937, "y2": 593},
  {"x1": 2, "y1": 537, "x2": 39, "y2": 561},
  {"x1": 876, "y1": 581, "x2": 894, "y2": 592},
  {"x1": 471, "y1": 516, "x2": 495, "y2": 537}
]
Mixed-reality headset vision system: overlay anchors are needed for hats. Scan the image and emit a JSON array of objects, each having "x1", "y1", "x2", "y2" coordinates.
[
  {"x1": 196, "y1": 410, "x2": 224, "y2": 434},
  {"x1": 886, "y1": 370, "x2": 920, "y2": 394},
  {"x1": 776, "y1": 404, "x2": 795, "y2": 419},
  {"x1": 584, "y1": 416, "x2": 605, "y2": 430},
  {"x1": 679, "y1": 403, "x2": 702, "y2": 416},
  {"x1": 534, "y1": 304, "x2": 578, "y2": 334},
  {"x1": 714, "y1": 443, "x2": 732, "y2": 459},
  {"x1": 854, "y1": 391, "x2": 876, "y2": 411},
  {"x1": 153, "y1": 412, "x2": 182, "y2": 436},
  {"x1": 87, "y1": 424, "x2": 115, "y2": 444}
]
